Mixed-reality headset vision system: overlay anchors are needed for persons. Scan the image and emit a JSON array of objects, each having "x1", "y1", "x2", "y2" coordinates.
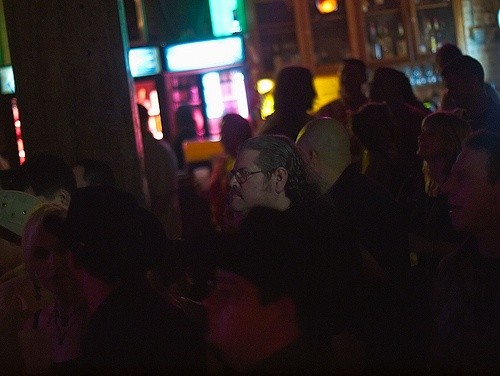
[{"x1": 0, "y1": 42, "x2": 500, "y2": 376}]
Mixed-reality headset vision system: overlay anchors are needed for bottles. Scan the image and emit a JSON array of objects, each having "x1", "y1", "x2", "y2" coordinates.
[
  {"x1": 367, "y1": 21, "x2": 410, "y2": 63},
  {"x1": 420, "y1": 16, "x2": 443, "y2": 54}
]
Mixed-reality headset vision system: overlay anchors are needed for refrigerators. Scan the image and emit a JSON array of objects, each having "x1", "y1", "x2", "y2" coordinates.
[
  {"x1": 162, "y1": 35, "x2": 254, "y2": 142},
  {"x1": 129, "y1": 44, "x2": 170, "y2": 158},
  {"x1": 0, "y1": 65, "x2": 26, "y2": 166}
]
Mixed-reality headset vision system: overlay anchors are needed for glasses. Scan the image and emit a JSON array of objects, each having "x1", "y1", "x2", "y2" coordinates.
[{"x1": 230, "y1": 168, "x2": 275, "y2": 184}]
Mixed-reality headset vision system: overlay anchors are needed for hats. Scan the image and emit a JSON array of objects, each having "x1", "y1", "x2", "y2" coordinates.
[{"x1": 43, "y1": 184, "x2": 161, "y2": 277}]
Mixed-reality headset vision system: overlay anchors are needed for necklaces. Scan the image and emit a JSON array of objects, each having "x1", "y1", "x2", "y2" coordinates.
[{"x1": 52, "y1": 305, "x2": 80, "y2": 344}]
